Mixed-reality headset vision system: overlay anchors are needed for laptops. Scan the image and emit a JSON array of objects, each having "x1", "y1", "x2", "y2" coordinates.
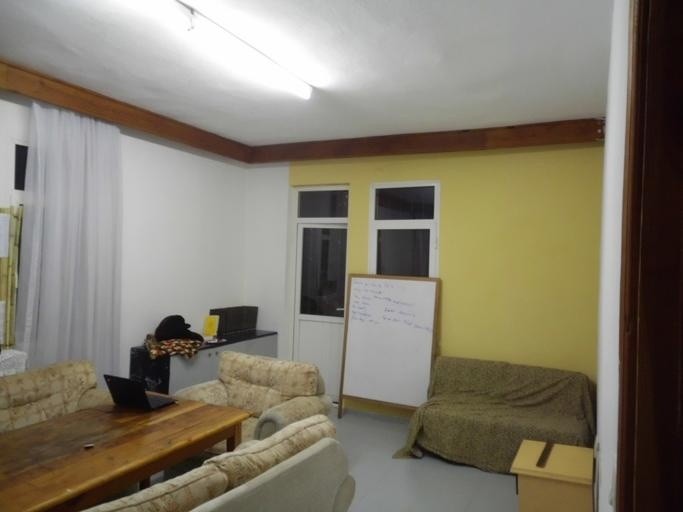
[{"x1": 103, "y1": 373, "x2": 175, "y2": 412}]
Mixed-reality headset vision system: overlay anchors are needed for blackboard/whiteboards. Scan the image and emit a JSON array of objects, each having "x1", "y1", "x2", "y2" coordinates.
[{"x1": 340, "y1": 273, "x2": 443, "y2": 409}]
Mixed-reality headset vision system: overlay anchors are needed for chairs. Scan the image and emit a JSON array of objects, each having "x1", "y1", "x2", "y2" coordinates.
[{"x1": 162, "y1": 351, "x2": 335, "y2": 481}]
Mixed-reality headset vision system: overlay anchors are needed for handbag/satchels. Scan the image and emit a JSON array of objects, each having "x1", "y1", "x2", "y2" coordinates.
[{"x1": 144, "y1": 315, "x2": 205, "y2": 361}]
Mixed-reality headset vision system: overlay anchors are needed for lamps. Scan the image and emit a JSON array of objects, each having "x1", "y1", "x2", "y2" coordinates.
[{"x1": 118, "y1": 0, "x2": 312, "y2": 101}]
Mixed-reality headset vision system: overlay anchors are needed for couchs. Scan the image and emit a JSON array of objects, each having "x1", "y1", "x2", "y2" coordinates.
[
  {"x1": 392, "y1": 355, "x2": 595, "y2": 476},
  {"x1": 0, "y1": 359, "x2": 114, "y2": 433},
  {"x1": 83, "y1": 413, "x2": 356, "y2": 512}
]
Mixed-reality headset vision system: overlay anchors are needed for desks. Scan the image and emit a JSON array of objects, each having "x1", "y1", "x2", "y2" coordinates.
[{"x1": 0, "y1": 389, "x2": 253, "y2": 512}]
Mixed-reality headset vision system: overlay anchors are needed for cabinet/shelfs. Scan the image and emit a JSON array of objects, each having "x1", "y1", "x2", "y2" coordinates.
[
  {"x1": 510, "y1": 439, "x2": 594, "y2": 512},
  {"x1": 130, "y1": 331, "x2": 278, "y2": 395}
]
